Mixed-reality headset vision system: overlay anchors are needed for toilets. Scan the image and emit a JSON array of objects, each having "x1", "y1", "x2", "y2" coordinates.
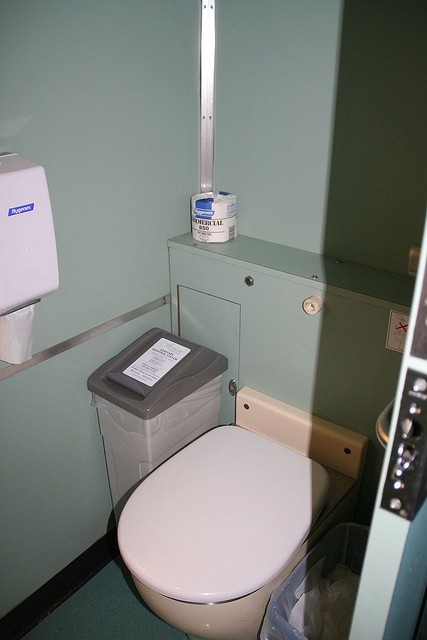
[{"x1": 116, "y1": 387, "x2": 370, "y2": 640}]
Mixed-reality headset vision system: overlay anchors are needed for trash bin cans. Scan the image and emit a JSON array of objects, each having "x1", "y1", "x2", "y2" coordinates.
[
  {"x1": 267, "y1": 522, "x2": 370, "y2": 640},
  {"x1": 87, "y1": 329, "x2": 228, "y2": 526}
]
